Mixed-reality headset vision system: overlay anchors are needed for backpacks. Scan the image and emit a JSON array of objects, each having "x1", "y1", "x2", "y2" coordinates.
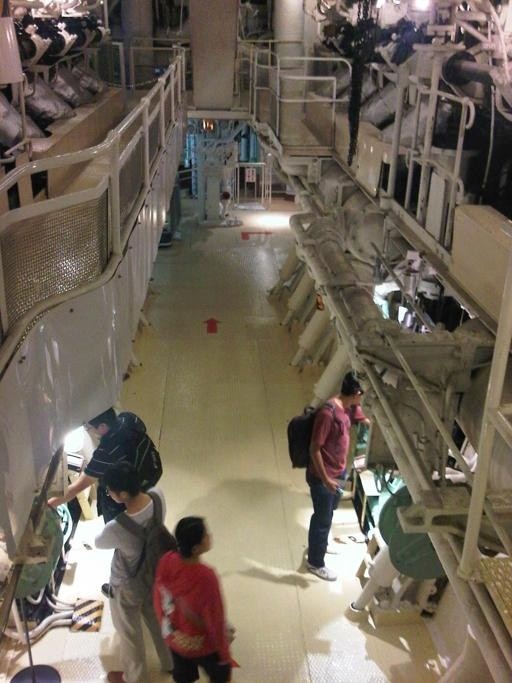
[
  {"x1": 116, "y1": 491, "x2": 177, "y2": 607},
  {"x1": 102, "y1": 419, "x2": 162, "y2": 490},
  {"x1": 288, "y1": 403, "x2": 335, "y2": 467}
]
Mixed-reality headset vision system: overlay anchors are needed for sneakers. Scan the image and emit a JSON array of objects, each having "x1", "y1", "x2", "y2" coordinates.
[{"x1": 307, "y1": 562, "x2": 336, "y2": 581}]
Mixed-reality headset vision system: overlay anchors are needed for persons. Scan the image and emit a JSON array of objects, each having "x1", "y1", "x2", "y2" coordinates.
[
  {"x1": 286, "y1": 370, "x2": 371, "y2": 582},
  {"x1": 44, "y1": 403, "x2": 163, "y2": 597},
  {"x1": 152, "y1": 515, "x2": 233, "y2": 683},
  {"x1": 93, "y1": 462, "x2": 173, "y2": 682}
]
